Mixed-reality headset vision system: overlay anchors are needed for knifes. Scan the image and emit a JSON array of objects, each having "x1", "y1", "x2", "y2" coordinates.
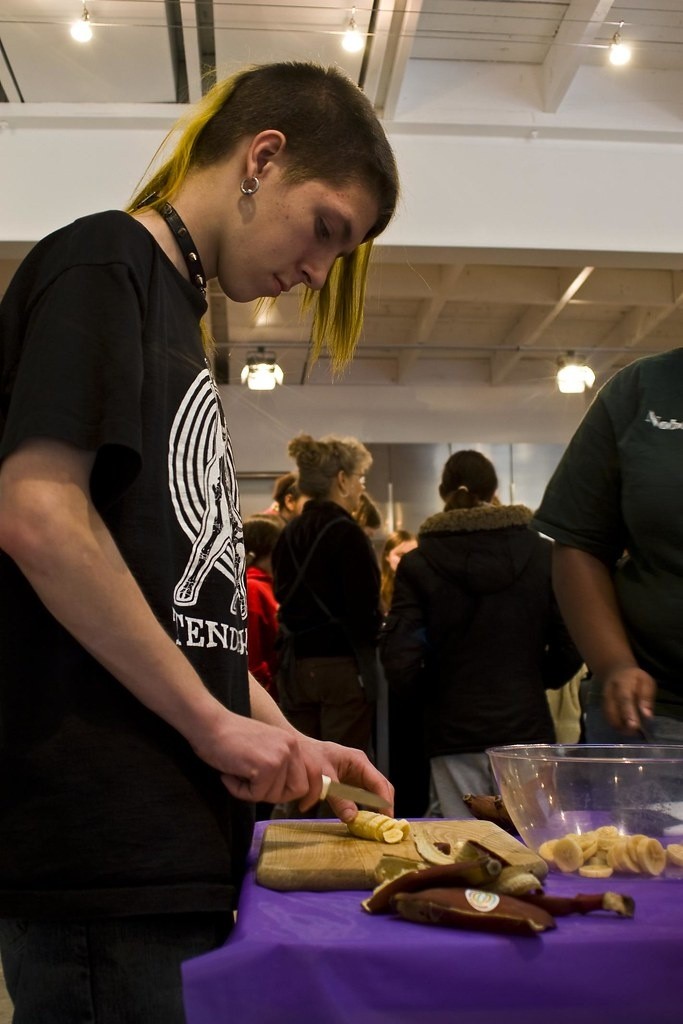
[{"x1": 318, "y1": 774, "x2": 391, "y2": 808}]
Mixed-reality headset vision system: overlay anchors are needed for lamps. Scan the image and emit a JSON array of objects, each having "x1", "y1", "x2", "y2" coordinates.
[
  {"x1": 555, "y1": 350, "x2": 596, "y2": 394},
  {"x1": 243, "y1": 349, "x2": 284, "y2": 390}
]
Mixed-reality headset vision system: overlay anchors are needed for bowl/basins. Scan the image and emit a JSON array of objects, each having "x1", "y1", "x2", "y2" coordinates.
[{"x1": 485, "y1": 745, "x2": 683, "y2": 879}]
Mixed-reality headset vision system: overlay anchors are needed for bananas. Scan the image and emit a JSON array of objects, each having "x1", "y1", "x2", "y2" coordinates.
[
  {"x1": 463, "y1": 763, "x2": 557, "y2": 825},
  {"x1": 538, "y1": 825, "x2": 683, "y2": 878},
  {"x1": 346, "y1": 810, "x2": 411, "y2": 844}
]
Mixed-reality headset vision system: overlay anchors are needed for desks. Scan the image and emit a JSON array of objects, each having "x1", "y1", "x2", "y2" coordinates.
[{"x1": 187, "y1": 810, "x2": 683, "y2": 1024}]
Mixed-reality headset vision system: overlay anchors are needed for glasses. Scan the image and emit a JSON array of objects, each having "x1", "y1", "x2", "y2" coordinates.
[{"x1": 352, "y1": 472, "x2": 367, "y2": 484}]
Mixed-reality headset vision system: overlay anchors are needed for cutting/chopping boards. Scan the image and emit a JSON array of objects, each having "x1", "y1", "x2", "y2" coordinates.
[{"x1": 256, "y1": 820, "x2": 549, "y2": 891}]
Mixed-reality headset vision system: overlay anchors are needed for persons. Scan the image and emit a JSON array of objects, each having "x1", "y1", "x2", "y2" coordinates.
[
  {"x1": 531, "y1": 345, "x2": 683, "y2": 810},
  {"x1": 243, "y1": 433, "x2": 589, "y2": 820},
  {"x1": 0, "y1": 61, "x2": 399, "y2": 1024}
]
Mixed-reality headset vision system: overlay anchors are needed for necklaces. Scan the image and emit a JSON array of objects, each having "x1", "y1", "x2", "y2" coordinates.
[{"x1": 133, "y1": 191, "x2": 207, "y2": 299}]
